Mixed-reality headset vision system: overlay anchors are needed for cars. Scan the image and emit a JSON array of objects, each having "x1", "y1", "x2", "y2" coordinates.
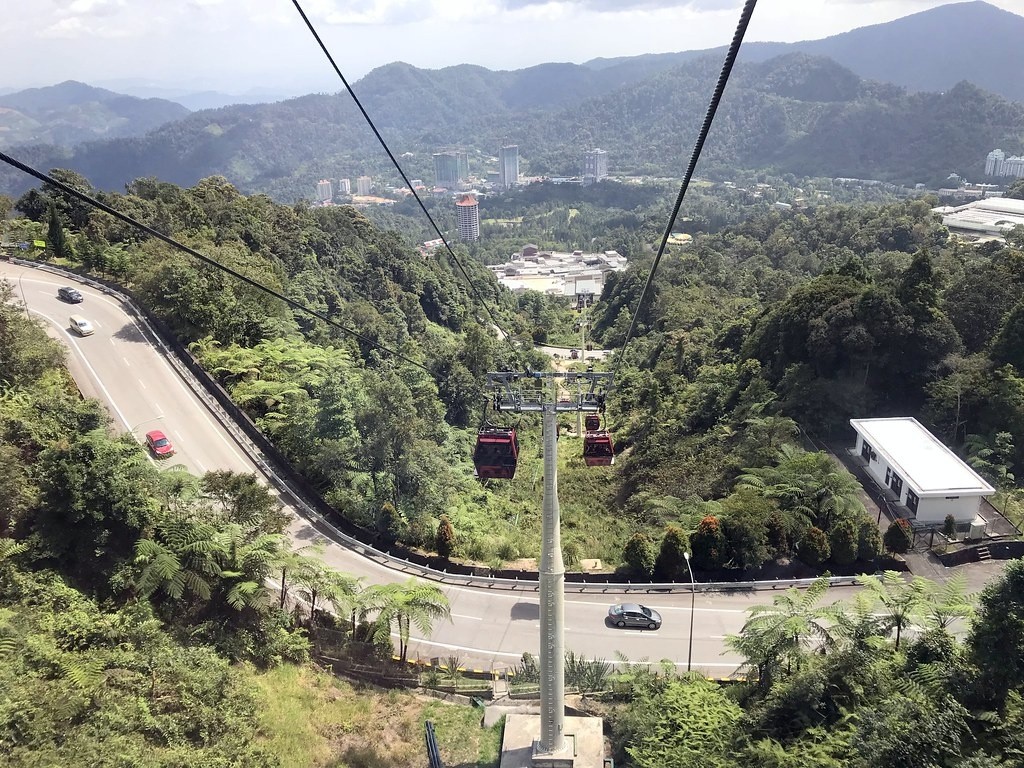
[
  {"x1": 608, "y1": 602, "x2": 662, "y2": 630},
  {"x1": 145, "y1": 430, "x2": 175, "y2": 459},
  {"x1": 58, "y1": 286, "x2": 83, "y2": 304}
]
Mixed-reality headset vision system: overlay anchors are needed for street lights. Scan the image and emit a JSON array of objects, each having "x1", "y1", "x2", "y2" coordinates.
[{"x1": 683, "y1": 551, "x2": 695, "y2": 683}]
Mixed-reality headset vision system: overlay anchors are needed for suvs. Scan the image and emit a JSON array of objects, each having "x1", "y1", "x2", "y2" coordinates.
[{"x1": 68, "y1": 314, "x2": 95, "y2": 337}]
